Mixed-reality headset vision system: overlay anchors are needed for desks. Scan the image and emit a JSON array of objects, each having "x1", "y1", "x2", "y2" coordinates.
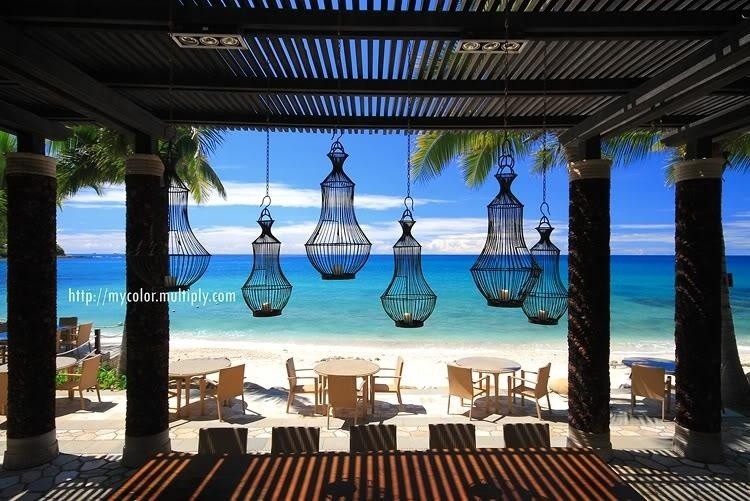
[
  {"x1": 455, "y1": 356, "x2": 521, "y2": 414},
  {"x1": 622, "y1": 358, "x2": 677, "y2": 409},
  {"x1": 167, "y1": 358, "x2": 231, "y2": 417},
  {"x1": 314, "y1": 359, "x2": 380, "y2": 417}
]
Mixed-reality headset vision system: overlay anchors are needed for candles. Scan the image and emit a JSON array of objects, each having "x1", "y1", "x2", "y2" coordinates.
[
  {"x1": 331, "y1": 264, "x2": 343, "y2": 275},
  {"x1": 538, "y1": 310, "x2": 548, "y2": 320},
  {"x1": 499, "y1": 289, "x2": 509, "y2": 301},
  {"x1": 263, "y1": 304, "x2": 272, "y2": 311},
  {"x1": 162, "y1": 276, "x2": 177, "y2": 286},
  {"x1": 403, "y1": 312, "x2": 413, "y2": 324}
]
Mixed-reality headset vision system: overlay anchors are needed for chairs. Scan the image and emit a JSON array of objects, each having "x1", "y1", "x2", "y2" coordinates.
[
  {"x1": 206, "y1": 364, "x2": 245, "y2": 422},
  {"x1": 504, "y1": 423, "x2": 549, "y2": 448},
  {"x1": 270, "y1": 427, "x2": 320, "y2": 454},
  {"x1": 198, "y1": 428, "x2": 247, "y2": 455},
  {"x1": 371, "y1": 355, "x2": 403, "y2": 414},
  {"x1": 508, "y1": 363, "x2": 552, "y2": 420},
  {"x1": 349, "y1": 424, "x2": 396, "y2": 452},
  {"x1": 447, "y1": 361, "x2": 490, "y2": 420},
  {"x1": 428, "y1": 424, "x2": 476, "y2": 448},
  {"x1": 327, "y1": 374, "x2": 365, "y2": 430},
  {"x1": 285, "y1": 358, "x2": 322, "y2": 414},
  {"x1": 630, "y1": 366, "x2": 671, "y2": 421},
  {"x1": 1, "y1": 316, "x2": 112, "y2": 417}
]
